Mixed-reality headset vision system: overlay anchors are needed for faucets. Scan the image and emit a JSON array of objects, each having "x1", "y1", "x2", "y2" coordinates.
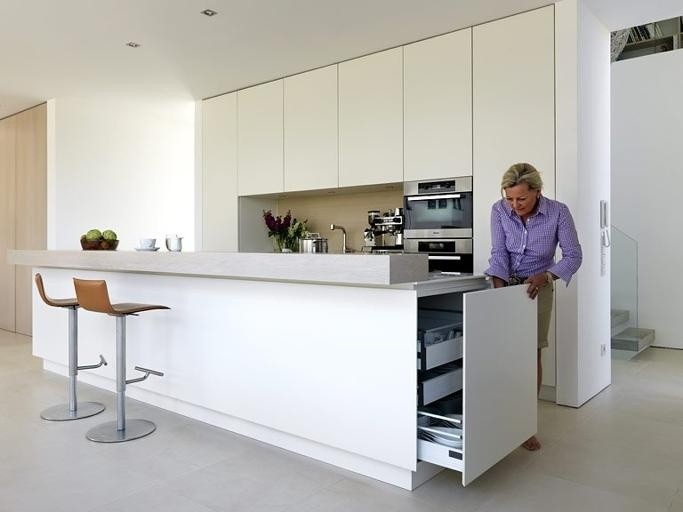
[{"x1": 330, "y1": 224, "x2": 354, "y2": 254}]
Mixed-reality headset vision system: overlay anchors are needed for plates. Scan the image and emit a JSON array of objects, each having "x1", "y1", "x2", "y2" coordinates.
[
  {"x1": 418, "y1": 412, "x2": 462, "y2": 448},
  {"x1": 134, "y1": 246, "x2": 160, "y2": 252}
]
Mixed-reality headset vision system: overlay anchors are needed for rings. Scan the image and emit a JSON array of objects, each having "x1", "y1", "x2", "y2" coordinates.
[{"x1": 536, "y1": 286, "x2": 539, "y2": 291}]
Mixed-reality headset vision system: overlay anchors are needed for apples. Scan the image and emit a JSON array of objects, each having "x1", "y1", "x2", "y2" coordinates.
[
  {"x1": 103, "y1": 230, "x2": 117, "y2": 241},
  {"x1": 86, "y1": 229, "x2": 101, "y2": 242}
]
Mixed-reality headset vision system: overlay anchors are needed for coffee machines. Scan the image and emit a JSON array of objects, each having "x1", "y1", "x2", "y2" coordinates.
[{"x1": 361, "y1": 210, "x2": 381, "y2": 248}]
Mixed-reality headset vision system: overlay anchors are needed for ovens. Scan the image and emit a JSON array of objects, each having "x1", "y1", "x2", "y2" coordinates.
[{"x1": 402, "y1": 175, "x2": 474, "y2": 274}]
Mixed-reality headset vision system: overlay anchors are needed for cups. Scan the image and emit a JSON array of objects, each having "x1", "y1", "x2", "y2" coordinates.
[{"x1": 165, "y1": 236, "x2": 183, "y2": 252}]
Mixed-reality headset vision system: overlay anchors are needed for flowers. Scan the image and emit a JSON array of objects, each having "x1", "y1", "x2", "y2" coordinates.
[{"x1": 260, "y1": 205, "x2": 313, "y2": 253}]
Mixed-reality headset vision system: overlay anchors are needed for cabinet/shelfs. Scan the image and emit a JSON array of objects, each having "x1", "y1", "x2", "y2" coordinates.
[
  {"x1": 199, "y1": 90, "x2": 279, "y2": 254},
  {"x1": 339, "y1": 25, "x2": 471, "y2": 196},
  {"x1": 417, "y1": 280, "x2": 539, "y2": 487},
  {"x1": 471, "y1": 2, "x2": 560, "y2": 388},
  {"x1": 236, "y1": 61, "x2": 339, "y2": 203}
]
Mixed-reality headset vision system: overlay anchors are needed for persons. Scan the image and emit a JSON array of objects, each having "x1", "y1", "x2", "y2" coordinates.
[{"x1": 483, "y1": 162, "x2": 582, "y2": 450}]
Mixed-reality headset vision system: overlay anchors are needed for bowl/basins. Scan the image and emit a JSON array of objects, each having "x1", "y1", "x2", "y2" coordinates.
[
  {"x1": 80, "y1": 238, "x2": 119, "y2": 251},
  {"x1": 139, "y1": 238, "x2": 157, "y2": 247}
]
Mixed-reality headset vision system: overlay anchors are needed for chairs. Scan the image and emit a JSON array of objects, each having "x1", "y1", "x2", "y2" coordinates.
[
  {"x1": 71, "y1": 276, "x2": 173, "y2": 444},
  {"x1": 32, "y1": 272, "x2": 107, "y2": 422}
]
